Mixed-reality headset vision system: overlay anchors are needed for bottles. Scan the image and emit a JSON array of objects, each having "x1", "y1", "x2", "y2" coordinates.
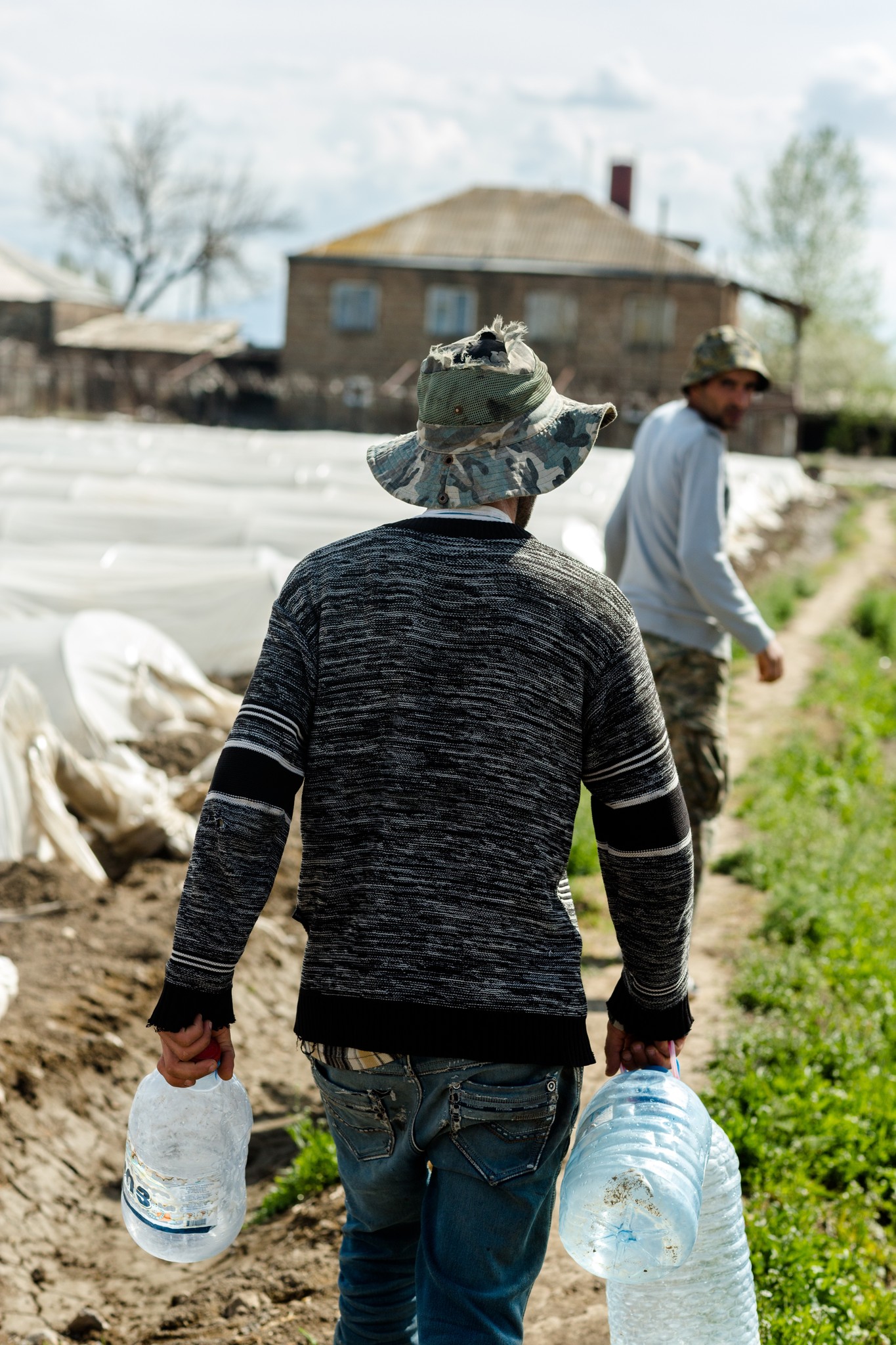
[
  {"x1": 120, "y1": 1037, "x2": 254, "y2": 1265},
  {"x1": 606, "y1": 1056, "x2": 759, "y2": 1345},
  {"x1": 557, "y1": 1039, "x2": 712, "y2": 1284}
]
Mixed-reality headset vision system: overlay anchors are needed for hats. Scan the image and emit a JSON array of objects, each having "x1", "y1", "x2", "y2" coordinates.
[
  {"x1": 367, "y1": 316, "x2": 618, "y2": 510},
  {"x1": 679, "y1": 324, "x2": 771, "y2": 392}
]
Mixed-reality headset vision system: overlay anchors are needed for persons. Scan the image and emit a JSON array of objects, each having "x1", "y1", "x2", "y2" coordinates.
[
  {"x1": 144, "y1": 310, "x2": 697, "y2": 1345},
  {"x1": 601, "y1": 323, "x2": 785, "y2": 1001}
]
